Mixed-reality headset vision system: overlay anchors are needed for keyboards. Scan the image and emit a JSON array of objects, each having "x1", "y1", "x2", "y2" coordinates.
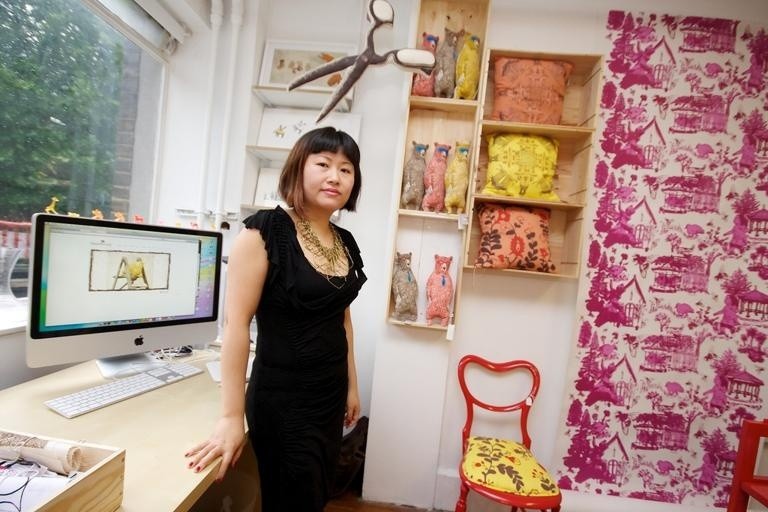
[{"x1": 44, "y1": 363, "x2": 202, "y2": 417}]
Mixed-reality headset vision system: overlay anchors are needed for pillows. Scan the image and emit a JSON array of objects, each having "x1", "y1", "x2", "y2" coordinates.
[
  {"x1": 472, "y1": 201, "x2": 557, "y2": 273},
  {"x1": 490, "y1": 54, "x2": 574, "y2": 125},
  {"x1": 481, "y1": 131, "x2": 565, "y2": 204}
]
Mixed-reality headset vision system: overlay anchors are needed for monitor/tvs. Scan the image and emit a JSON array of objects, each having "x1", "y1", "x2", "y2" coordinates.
[{"x1": 25, "y1": 211, "x2": 222, "y2": 377}]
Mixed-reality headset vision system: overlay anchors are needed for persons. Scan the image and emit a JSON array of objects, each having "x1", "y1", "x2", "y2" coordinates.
[{"x1": 185, "y1": 126, "x2": 368, "y2": 510}]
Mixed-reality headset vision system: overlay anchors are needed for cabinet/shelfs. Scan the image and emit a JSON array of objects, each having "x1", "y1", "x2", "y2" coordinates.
[
  {"x1": 459, "y1": 47, "x2": 605, "y2": 281},
  {"x1": 238, "y1": 78, "x2": 349, "y2": 224},
  {"x1": 380, "y1": 1, "x2": 496, "y2": 333}
]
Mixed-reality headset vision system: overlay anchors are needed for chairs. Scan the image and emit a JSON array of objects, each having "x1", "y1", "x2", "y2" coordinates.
[
  {"x1": 725, "y1": 412, "x2": 768, "y2": 512},
  {"x1": 452, "y1": 355, "x2": 565, "y2": 512}
]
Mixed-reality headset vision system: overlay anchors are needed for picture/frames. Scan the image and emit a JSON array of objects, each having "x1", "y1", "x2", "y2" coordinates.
[{"x1": 258, "y1": 37, "x2": 359, "y2": 103}]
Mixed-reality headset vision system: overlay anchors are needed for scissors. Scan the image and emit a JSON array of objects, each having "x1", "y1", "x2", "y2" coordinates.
[{"x1": 289, "y1": 0, "x2": 437, "y2": 124}]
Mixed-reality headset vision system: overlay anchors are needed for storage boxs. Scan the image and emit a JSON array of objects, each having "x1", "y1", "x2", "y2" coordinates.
[{"x1": 0, "y1": 429, "x2": 126, "y2": 511}]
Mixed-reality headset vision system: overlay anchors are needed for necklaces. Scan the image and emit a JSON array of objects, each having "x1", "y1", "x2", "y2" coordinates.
[{"x1": 296, "y1": 208, "x2": 354, "y2": 289}]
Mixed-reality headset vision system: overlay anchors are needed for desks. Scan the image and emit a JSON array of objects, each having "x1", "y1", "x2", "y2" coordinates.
[{"x1": 0, "y1": 347, "x2": 256, "y2": 511}]
